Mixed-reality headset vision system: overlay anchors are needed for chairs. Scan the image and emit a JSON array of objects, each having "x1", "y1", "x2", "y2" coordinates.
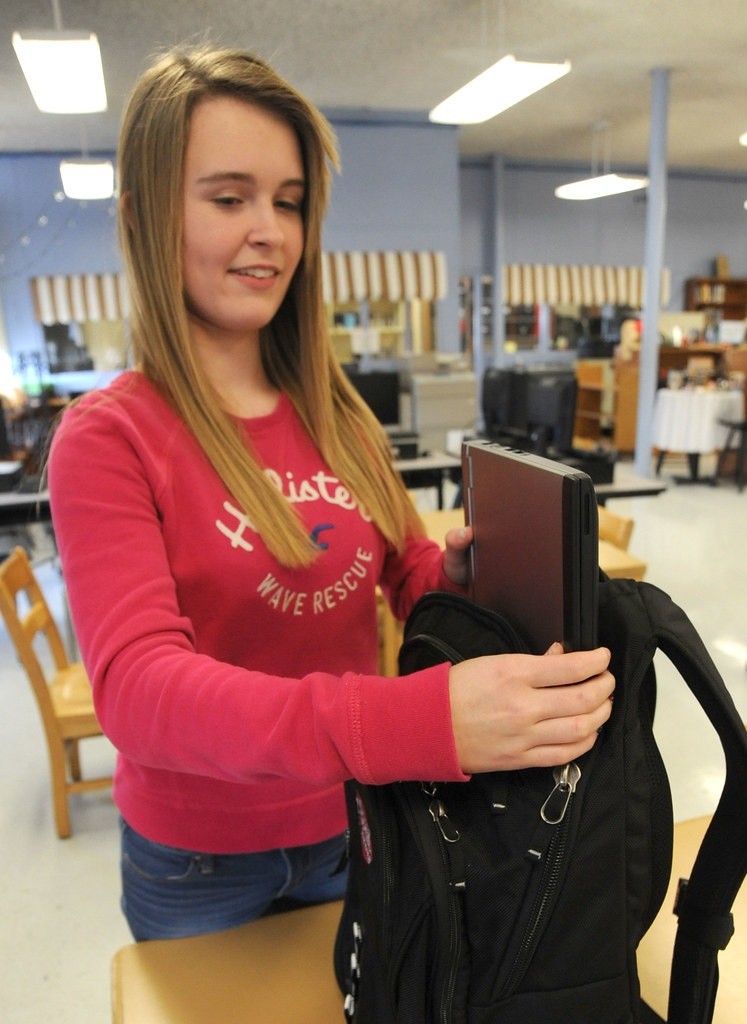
[{"x1": 2, "y1": 545, "x2": 119, "y2": 837}]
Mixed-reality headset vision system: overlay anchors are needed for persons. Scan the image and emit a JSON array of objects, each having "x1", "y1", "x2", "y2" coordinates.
[{"x1": 44, "y1": 41, "x2": 620, "y2": 941}]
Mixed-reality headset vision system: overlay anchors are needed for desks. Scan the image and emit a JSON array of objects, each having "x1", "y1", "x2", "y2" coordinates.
[
  {"x1": 101, "y1": 814, "x2": 744, "y2": 1023},
  {"x1": 654, "y1": 382, "x2": 743, "y2": 484}
]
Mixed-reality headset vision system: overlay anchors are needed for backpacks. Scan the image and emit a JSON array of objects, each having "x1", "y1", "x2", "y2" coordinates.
[{"x1": 328, "y1": 561, "x2": 747, "y2": 1024}]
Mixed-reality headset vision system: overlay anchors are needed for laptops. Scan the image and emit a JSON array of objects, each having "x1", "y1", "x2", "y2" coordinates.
[{"x1": 460, "y1": 439, "x2": 599, "y2": 656}]
